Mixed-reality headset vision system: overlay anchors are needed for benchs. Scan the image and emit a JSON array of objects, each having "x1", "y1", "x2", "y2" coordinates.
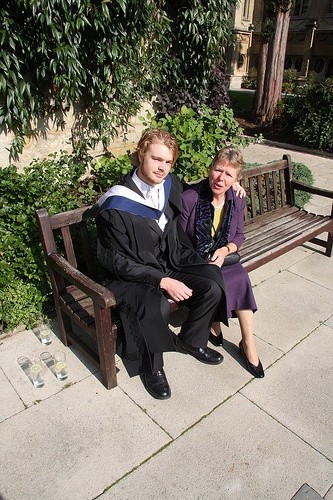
[{"x1": 36, "y1": 153, "x2": 333, "y2": 391}]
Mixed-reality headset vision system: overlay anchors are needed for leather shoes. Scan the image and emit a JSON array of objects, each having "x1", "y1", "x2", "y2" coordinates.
[
  {"x1": 143, "y1": 357, "x2": 171, "y2": 399},
  {"x1": 175, "y1": 332, "x2": 223, "y2": 365},
  {"x1": 208, "y1": 330, "x2": 224, "y2": 346},
  {"x1": 239, "y1": 340, "x2": 265, "y2": 378}
]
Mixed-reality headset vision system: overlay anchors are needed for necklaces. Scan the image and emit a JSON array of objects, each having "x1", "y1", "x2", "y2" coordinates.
[{"x1": 214, "y1": 201, "x2": 225, "y2": 209}]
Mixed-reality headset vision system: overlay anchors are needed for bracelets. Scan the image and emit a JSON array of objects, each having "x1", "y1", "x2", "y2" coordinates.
[{"x1": 225, "y1": 245, "x2": 229, "y2": 252}]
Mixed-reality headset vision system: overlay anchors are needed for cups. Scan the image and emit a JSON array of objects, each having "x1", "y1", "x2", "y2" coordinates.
[
  {"x1": 53, "y1": 350, "x2": 68, "y2": 381},
  {"x1": 29, "y1": 357, "x2": 44, "y2": 388},
  {"x1": 39, "y1": 319, "x2": 52, "y2": 346}
]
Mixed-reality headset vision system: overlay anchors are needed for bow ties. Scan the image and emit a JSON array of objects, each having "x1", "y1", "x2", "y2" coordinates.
[{"x1": 147, "y1": 179, "x2": 165, "y2": 200}]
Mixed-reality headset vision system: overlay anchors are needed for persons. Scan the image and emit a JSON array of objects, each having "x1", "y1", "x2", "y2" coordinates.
[
  {"x1": 178, "y1": 146, "x2": 265, "y2": 378},
  {"x1": 94, "y1": 129, "x2": 246, "y2": 400}
]
